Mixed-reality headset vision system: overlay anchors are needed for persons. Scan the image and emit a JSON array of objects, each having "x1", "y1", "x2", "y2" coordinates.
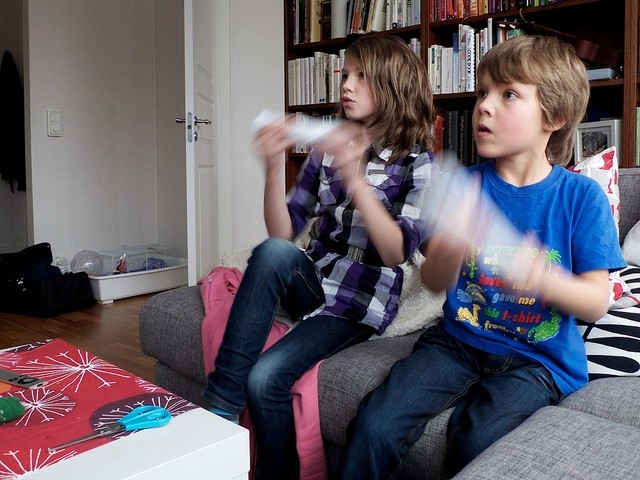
[
  {"x1": 205, "y1": 33, "x2": 440, "y2": 480},
  {"x1": 339, "y1": 35, "x2": 627, "y2": 479}
]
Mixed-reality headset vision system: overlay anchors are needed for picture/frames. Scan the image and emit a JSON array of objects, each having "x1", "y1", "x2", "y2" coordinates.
[{"x1": 574, "y1": 120, "x2": 622, "y2": 167}]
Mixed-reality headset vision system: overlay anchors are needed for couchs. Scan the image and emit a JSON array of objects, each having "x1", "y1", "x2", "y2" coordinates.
[{"x1": 140, "y1": 169, "x2": 640, "y2": 480}]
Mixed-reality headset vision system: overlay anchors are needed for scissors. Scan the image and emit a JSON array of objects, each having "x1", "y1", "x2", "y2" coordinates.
[{"x1": 46, "y1": 405, "x2": 170, "y2": 452}]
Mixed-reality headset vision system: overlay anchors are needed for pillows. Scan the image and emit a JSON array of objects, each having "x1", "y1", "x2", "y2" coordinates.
[
  {"x1": 565, "y1": 146, "x2": 620, "y2": 242},
  {"x1": 619, "y1": 219, "x2": 640, "y2": 266}
]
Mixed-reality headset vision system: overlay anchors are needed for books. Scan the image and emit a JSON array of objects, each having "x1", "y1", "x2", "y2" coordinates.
[
  {"x1": 291, "y1": 0, "x2": 421, "y2": 46},
  {"x1": 406, "y1": 36, "x2": 422, "y2": 59},
  {"x1": 430, "y1": 0, "x2": 559, "y2": 23},
  {"x1": 428, "y1": 18, "x2": 524, "y2": 95},
  {"x1": 289, "y1": 111, "x2": 338, "y2": 155},
  {"x1": 431, "y1": 99, "x2": 490, "y2": 168},
  {"x1": 287, "y1": 51, "x2": 345, "y2": 105}
]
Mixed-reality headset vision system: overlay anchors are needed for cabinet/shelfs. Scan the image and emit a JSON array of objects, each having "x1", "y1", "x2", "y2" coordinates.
[{"x1": 283, "y1": 1, "x2": 638, "y2": 193}]
